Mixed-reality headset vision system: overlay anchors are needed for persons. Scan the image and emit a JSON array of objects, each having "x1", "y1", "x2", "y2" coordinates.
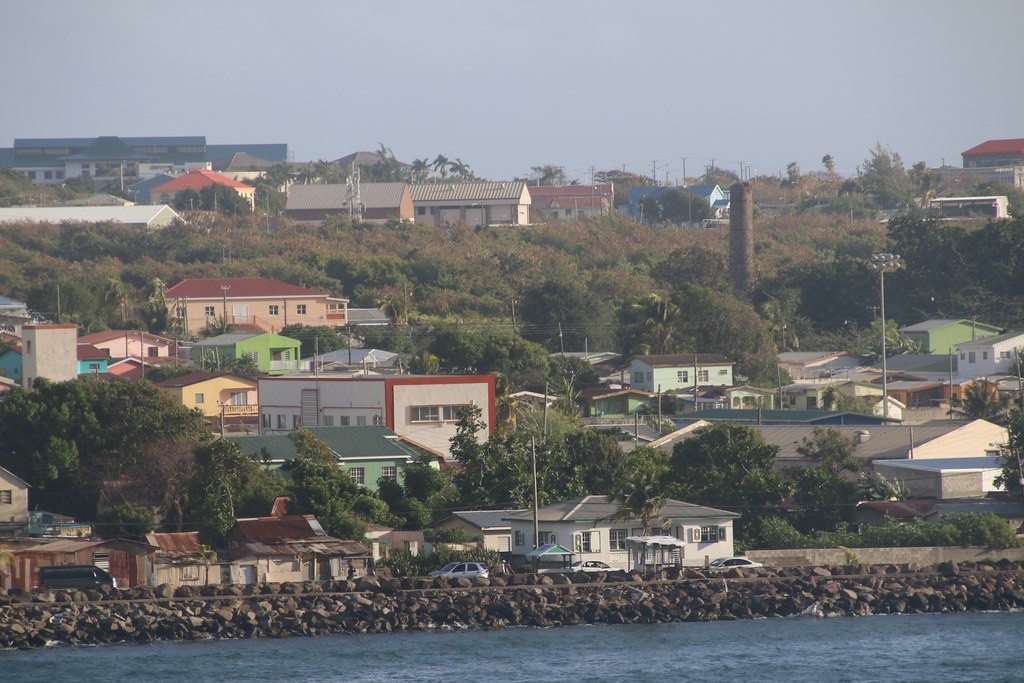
[
  {"x1": 348, "y1": 565, "x2": 356, "y2": 580},
  {"x1": 498, "y1": 560, "x2": 515, "y2": 574}
]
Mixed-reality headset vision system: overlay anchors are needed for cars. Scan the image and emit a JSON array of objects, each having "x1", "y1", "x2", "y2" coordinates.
[
  {"x1": 428, "y1": 561, "x2": 490, "y2": 579},
  {"x1": 560, "y1": 560, "x2": 621, "y2": 572},
  {"x1": 703, "y1": 556, "x2": 763, "y2": 573}
]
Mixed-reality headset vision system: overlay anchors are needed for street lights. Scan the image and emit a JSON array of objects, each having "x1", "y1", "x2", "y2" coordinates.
[{"x1": 866, "y1": 253, "x2": 901, "y2": 424}]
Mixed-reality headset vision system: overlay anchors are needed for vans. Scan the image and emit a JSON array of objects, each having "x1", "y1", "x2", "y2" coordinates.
[{"x1": 40, "y1": 565, "x2": 114, "y2": 594}]
